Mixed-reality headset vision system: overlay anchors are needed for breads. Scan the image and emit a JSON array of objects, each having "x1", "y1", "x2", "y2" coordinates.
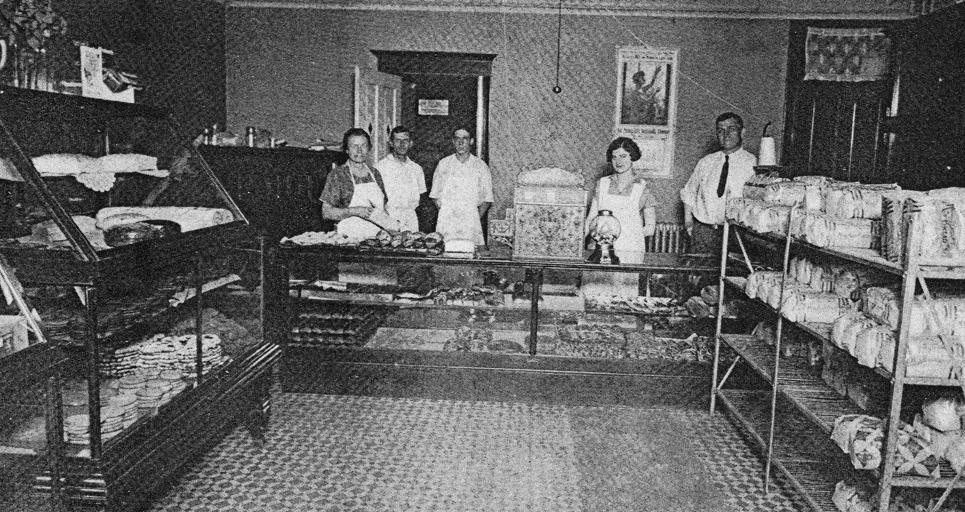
[
  {"x1": 376, "y1": 230, "x2": 443, "y2": 248},
  {"x1": 456, "y1": 324, "x2": 700, "y2": 360},
  {"x1": 288, "y1": 301, "x2": 399, "y2": 349},
  {"x1": 96, "y1": 212, "x2": 151, "y2": 232},
  {"x1": 724, "y1": 174, "x2": 965, "y2": 507}
]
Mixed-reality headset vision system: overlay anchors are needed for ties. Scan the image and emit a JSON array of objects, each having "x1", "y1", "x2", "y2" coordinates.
[{"x1": 717, "y1": 156, "x2": 728, "y2": 198}]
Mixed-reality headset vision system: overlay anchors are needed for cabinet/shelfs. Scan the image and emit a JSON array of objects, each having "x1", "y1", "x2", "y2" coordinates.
[
  {"x1": 265, "y1": 242, "x2": 772, "y2": 384},
  {"x1": 703, "y1": 167, "x2": 963, "y2": 512},
  {"x1": 0, "y1": 86, "x2": 282, "y2": 511}
]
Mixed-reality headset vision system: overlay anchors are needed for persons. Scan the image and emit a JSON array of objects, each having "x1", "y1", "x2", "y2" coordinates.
[
  {"x1": 679, "y1": 111, "x2": 757, "y2": 254},
  {"x1": 371, "y1": 124, "x2": 427, "y2": 236},
  {"x1": 428, "y1": 125, "x2": 494, "y2": 247},
  {"x1": 628, "y1": 65, "x2": 665, "y2": 126},
  {"x1": 320, "y1": 126, "x2": 391, "y2": 238},
  {"x1": 581, "y1": 135, "x2": 662, "y2": 299}
]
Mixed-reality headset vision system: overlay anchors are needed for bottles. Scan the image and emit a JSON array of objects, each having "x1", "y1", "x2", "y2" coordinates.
[
  {"x1": 245, "y1": 126, "x2": 257, "y2": 148},
  {"x1": 203, "y1": 124, "x2": 217, "y2": 146},
  {"x1": 647, "y1": 221, "x2": 687, "y2": 254}
]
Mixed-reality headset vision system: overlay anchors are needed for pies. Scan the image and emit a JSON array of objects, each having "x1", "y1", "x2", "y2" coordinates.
[{"x1": 5, "y1": 333, "x2": 224, "y2": 444}]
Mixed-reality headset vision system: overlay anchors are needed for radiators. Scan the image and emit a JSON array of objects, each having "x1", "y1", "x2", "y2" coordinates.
[{"x1": 644, "y1": 222, "x2": 684, "y2": 255}]
